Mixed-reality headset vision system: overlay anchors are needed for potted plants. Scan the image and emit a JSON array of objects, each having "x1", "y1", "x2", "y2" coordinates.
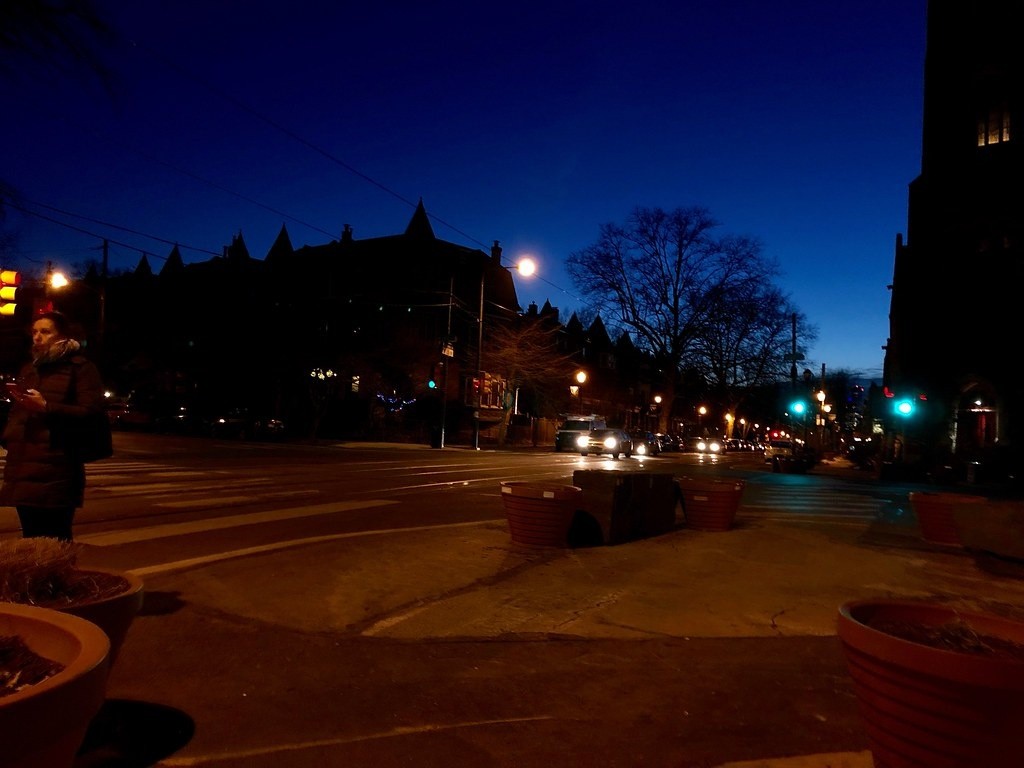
[{"x1": 0, "y1": 533, "x2": 144, "y2": 678}]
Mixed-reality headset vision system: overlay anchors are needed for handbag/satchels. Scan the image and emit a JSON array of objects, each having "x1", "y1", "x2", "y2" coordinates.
[{"x1": 41, "y1": 359, "x2": 112, "y2": 464}]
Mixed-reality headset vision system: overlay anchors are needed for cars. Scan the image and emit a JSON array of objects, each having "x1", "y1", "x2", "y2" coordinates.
[
  {"x1": 629, "y1": 430, "x2": 659, "y2": 455},
  {"x1": 574, "y1": 426, "x2": 633, "y2": 458},
  {"x1": 763, "y1": 440, "x2": 792, "y2": 461},
  {"x1": 107, "y1": 401, "x2": 150, "y2": 431},
  {"x1": 654, "y1": 434, "x2": 764, "y2": 452},
  {"x1": 151, "y1": 400, "x2": 285, "y2": 440}
]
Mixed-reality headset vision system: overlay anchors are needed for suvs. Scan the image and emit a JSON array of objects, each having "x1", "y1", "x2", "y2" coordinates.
[{"x1": 554, "y1": 420, "x2": 606, "y2": 450}]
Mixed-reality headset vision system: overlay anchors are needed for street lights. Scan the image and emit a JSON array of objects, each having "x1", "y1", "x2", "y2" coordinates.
[
  {"x1": 472, "y1": 252, "x2": 543, "y2": 450},
  {"x1": 698, "y1": 407, "x2": 706, "y2": 434},
  {"x1": 654, "y1": 396, "x2": 662, "y2": 432},
  {"x1": 803, "y1": 368, "x2": 811, "y2": 453},
  {"x1": 725, "y1": 414, "x2": 770, "y2": 442},
  {"x1": 576, "y1": 370, "x2": 588, "y2": 417}
]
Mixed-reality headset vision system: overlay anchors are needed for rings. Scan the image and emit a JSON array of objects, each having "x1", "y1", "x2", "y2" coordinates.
[{"x1": 21, "y1": 399, "x2": 23, "y2": 401}]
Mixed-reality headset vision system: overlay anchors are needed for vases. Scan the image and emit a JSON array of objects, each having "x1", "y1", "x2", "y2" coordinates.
[
  {"x1": 835, "y1": 594, "x2": 1024, "y2": 768},
  {"x1": 673, "y1": 474, "x2": 745, "y2": 533},
  {"x1": 500, "y1": 480, "x2": 583, "y2": 551},
  {"x1": 907, "y1": 491, "x2": 988, "y2": 549},
  {"x1": 0, "y1": 599, "x2": 111, "y2": 768}
]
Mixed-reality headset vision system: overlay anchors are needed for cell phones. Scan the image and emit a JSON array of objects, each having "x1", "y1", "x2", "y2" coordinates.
[{"x1": 6, "y1": 382, "x2": 20, "y2": 394}]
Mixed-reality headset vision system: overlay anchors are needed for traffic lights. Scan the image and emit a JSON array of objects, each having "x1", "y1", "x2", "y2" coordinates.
[
  {"x1": 478, "y1": 370, "x2": 493, "y2": 394},
  {"x1": 883, "y1": 383, "x2": 929, "y2": 422},
  {"x1": 428, "y1": 367, "x2": 442, "y2": 388},
  {"x1": 790, "y1": 400, "x2": 807, "y2": 418},
  {"x1": 0, "y1": 268, "x2": 20, "y2": 319}
]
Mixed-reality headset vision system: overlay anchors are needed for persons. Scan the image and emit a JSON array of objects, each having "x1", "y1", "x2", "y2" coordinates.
[{"x1": 0, "y1": 311, "x2": 100, "y2": 540}]
